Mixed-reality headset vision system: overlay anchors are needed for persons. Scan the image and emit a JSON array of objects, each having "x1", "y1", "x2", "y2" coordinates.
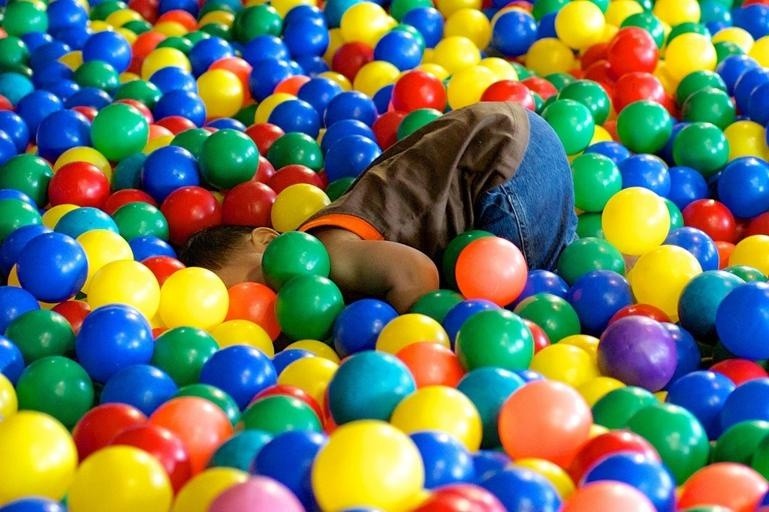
[{"x1": 178, "y1": 100, "x2": 576, "y2": 315}]
